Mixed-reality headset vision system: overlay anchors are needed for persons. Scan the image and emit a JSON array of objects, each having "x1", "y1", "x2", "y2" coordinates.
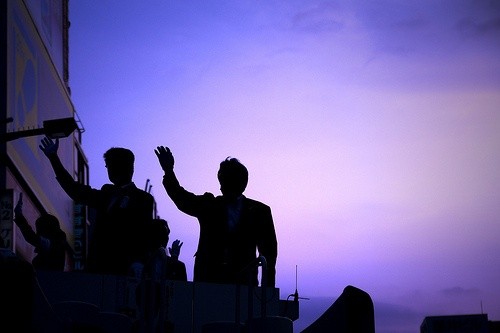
[
  {"x1": 154, "y1": 143, "x2": 279, "y2": 289},
  {"x1": 14, "y1": 189, "x2": 66, "y2": 284},
  {"x1": 43, "y1": 132, "x2": 156, "y2": 278},
  {"x1": 155, "y1": 215, "x2": 188, "y2": 283}
]
